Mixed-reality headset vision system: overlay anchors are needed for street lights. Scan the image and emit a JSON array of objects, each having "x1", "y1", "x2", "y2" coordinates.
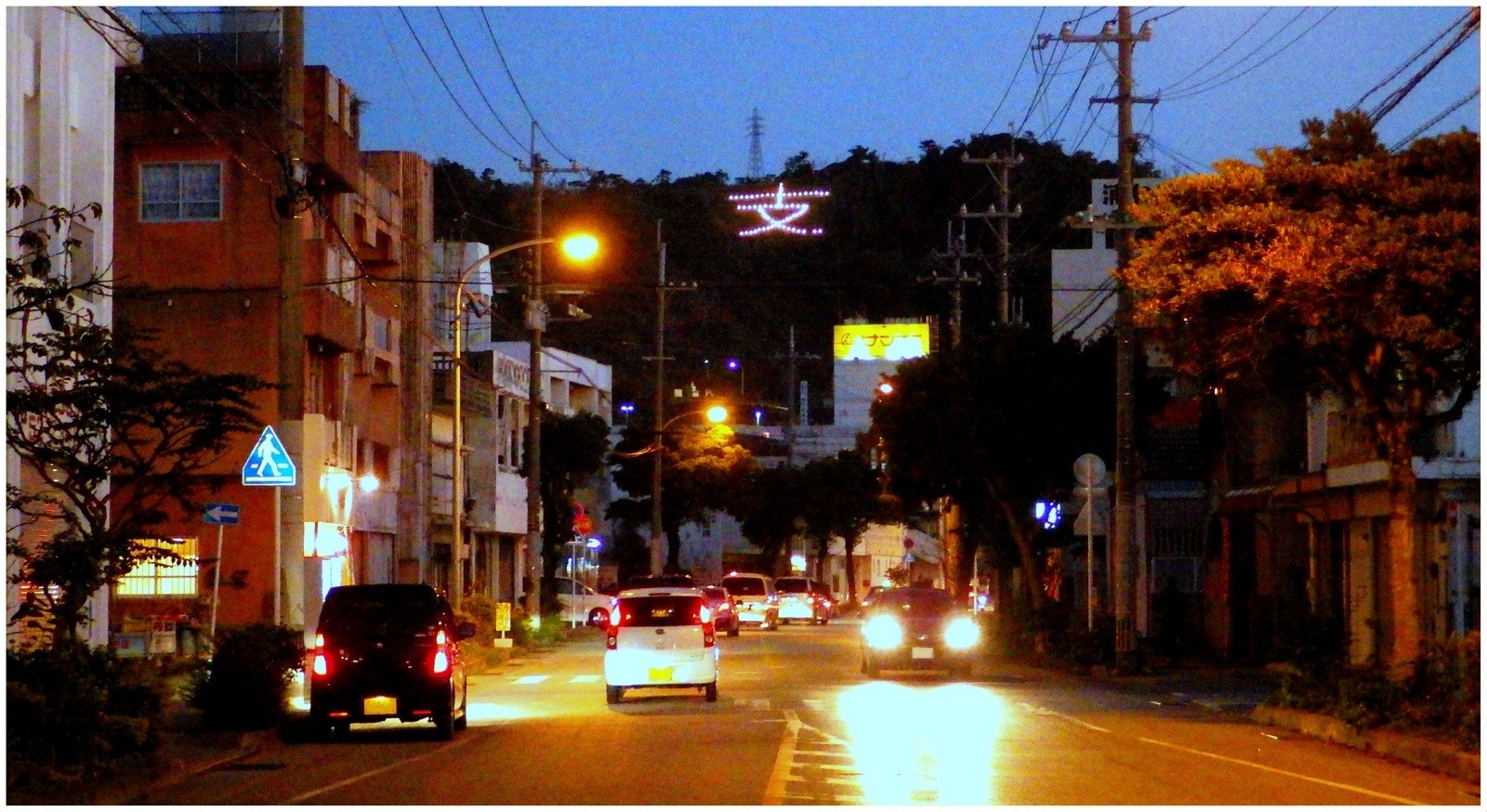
[
  {"x1": 654, "y1": 403, "x2": 729, "y2": 574},
  {"x1": 451, "y1": 228, "x2": 609, "y2": 620}
]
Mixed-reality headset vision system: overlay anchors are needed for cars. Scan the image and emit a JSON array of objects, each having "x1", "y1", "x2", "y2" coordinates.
[
  {"x1": 855, "y1": 587, "x2": 980, "y2": 680},
  {"x1": 859, "y1": 585, "x2": 889, "y2": 613},
  {"x1": 554, "y1": 575, "x2": 617, "y2": 627},
  {"x1": 968, "y1": 584, "x2": 980, "y2": 608},
  {"x1": 695, "y1": 585, "x2": 744, "y2": 637}
]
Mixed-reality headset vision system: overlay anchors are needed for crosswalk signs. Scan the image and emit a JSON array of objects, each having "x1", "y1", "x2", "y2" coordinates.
[{"x1": 242, "y1": 424, "x2": 296, "y2": 486}]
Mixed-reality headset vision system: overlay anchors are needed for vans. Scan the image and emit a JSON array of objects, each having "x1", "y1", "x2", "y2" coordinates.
[
  {"x1": 714, "y1": 573, "x2": 783, "y2": 633},
  {"x1": 774, "y1": 576, "x2": 835, "y2": 627}
]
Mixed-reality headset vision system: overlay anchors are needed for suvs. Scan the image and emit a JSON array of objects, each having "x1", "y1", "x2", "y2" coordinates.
[
  {"x1": 308, "y1": 582, "x2": 477, "y2": 741},
  {"x1": 596, "y1": 575, "x2": 717, "y2": 705}
]
